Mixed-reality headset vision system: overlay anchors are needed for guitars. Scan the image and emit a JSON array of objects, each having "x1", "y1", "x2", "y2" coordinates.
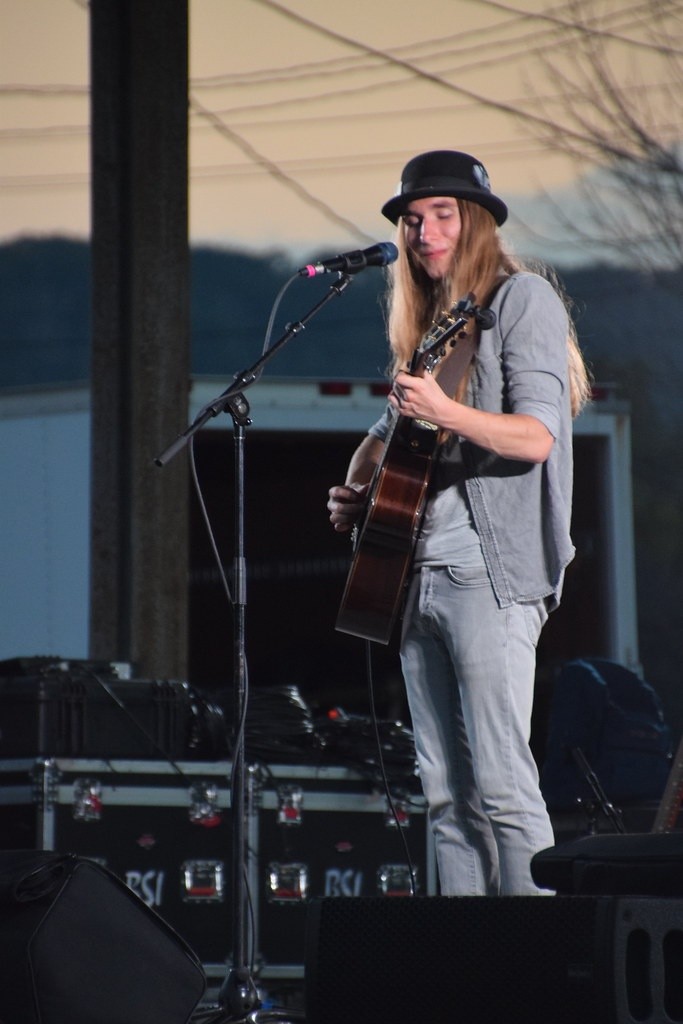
[{"x1": 336, "y1": 291, "x2": 497, "y2": 646}]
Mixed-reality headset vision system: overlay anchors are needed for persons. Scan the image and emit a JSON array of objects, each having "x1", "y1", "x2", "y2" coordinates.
[{"x1": 321, "y1": 148, "x2": 580, "y2": 895}]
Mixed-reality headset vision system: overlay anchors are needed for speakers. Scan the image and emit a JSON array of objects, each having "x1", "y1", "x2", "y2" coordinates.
[
  {"x1": 304, "y1": 895, "x2": 682, "y2": 1024},
  {"x1": 1, "y1": 848, "x2": 206, "y2": 1024}
]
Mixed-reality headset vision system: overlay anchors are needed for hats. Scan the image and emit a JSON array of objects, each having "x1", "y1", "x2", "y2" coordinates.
[{"x1": 382, "y1": 150, "x2": 507, "y2": 229}]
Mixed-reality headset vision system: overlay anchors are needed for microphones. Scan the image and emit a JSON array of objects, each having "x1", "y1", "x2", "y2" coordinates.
[{"x1": 299, "y1": 242, "x2": 398, "y2": 276}]
